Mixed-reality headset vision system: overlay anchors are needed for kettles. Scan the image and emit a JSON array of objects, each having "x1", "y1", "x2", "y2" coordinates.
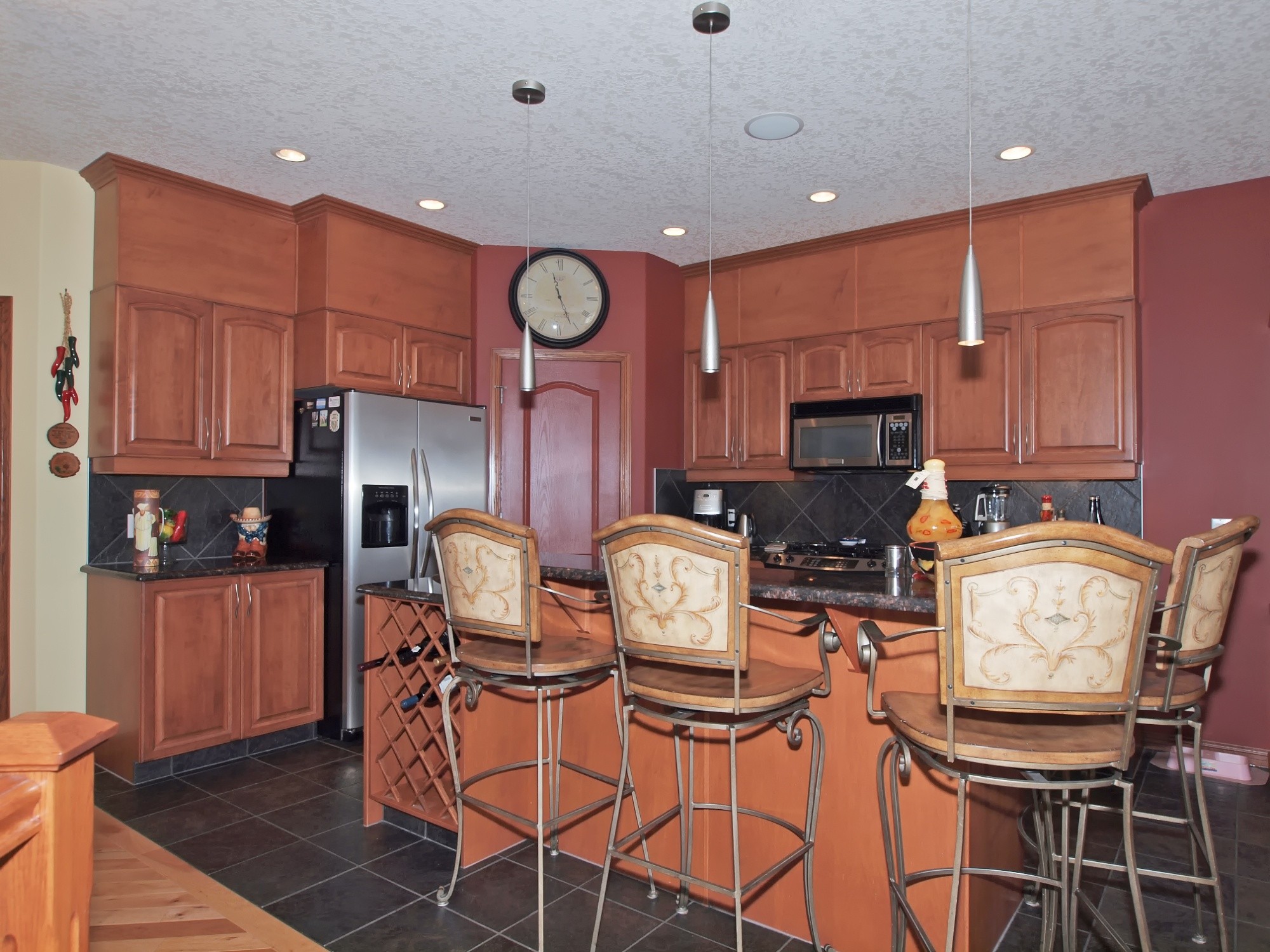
[{"x1": 733, "y1": 513, "x2": 759, "y2": 551}]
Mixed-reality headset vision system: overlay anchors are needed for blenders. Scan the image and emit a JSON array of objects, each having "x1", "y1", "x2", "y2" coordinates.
[{"x1": 974, "y1": 484, "x2": 1012, "y2": 537}]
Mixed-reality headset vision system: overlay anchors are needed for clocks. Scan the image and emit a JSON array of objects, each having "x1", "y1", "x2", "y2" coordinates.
[{"x1": 507, "y1": 247, "x2": 611, "y2": 350}]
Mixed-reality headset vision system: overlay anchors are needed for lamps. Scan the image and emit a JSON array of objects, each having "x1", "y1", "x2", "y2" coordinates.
[
  {"x1": 690, "y1": 1, "x2": 731, "y2": 374},
  {"x1": 956, "y1": 3, "x2": 987, "y2": 350},
  {"x1": 511, "y1": 81, "x2": 546, "y2": 394}
]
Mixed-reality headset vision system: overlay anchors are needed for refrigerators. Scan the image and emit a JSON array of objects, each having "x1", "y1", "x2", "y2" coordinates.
[{"x1": 287, "y1": 386, "x2": 485, "y2": 737}]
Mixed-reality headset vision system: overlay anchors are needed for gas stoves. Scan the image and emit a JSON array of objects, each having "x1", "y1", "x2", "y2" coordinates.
[{"x1": 764, "y1": 537, "x2": 888, "y2": 573}]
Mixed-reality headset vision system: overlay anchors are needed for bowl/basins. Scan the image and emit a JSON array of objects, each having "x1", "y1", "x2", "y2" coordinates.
[{"x1": 910, "y1": 542, "x2": 935, "y2": 574}]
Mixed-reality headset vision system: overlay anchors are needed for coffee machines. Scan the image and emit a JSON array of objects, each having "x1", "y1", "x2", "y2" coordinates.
[{"x1": 691, "y1": 482, "x2": 728, "y2": 533}]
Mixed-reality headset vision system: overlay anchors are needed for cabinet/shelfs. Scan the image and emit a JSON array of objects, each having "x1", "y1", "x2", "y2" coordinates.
[{"x1": 87, "y1": 283, "x2": 332, "y2": 787}]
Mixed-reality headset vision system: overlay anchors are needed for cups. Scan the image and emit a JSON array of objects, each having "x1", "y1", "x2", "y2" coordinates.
[
  {"x1": 885, "y1": 544, "x2": 906, "y2": 577},
  {"x1": 133, "y1": 489, "x2": 160, "y2": 566}
]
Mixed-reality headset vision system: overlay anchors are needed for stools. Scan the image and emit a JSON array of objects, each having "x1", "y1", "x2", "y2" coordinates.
[
  {"x1": 857, "y1": 520, "x2": 1152, "y2": 952},
  {"x1": 426, "y1": 507, "x2": 656, "y2": 951},
  {"x1": 1017, "y1": 517, "x2": 1261, "y2": 952},
  {"x1": 591, "y1": 513, "x2": 844, "y2": 951}
]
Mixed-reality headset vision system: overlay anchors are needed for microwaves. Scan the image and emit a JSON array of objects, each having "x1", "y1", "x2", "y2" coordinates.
[{"x1": 787, "y1": 394, "x2": 923, "y2": 475}]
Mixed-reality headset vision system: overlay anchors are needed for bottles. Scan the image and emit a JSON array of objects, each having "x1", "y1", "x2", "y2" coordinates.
[
  {"x1": 1086, "y1": 493, "x2": 1104, "y2": 525},
  {"x1": 1058, "y1": 509, "x2": 1064, "y2": 521},
  {"x1": 1040, "y1": 494, "x2": 1054, "y2": 521}
]
